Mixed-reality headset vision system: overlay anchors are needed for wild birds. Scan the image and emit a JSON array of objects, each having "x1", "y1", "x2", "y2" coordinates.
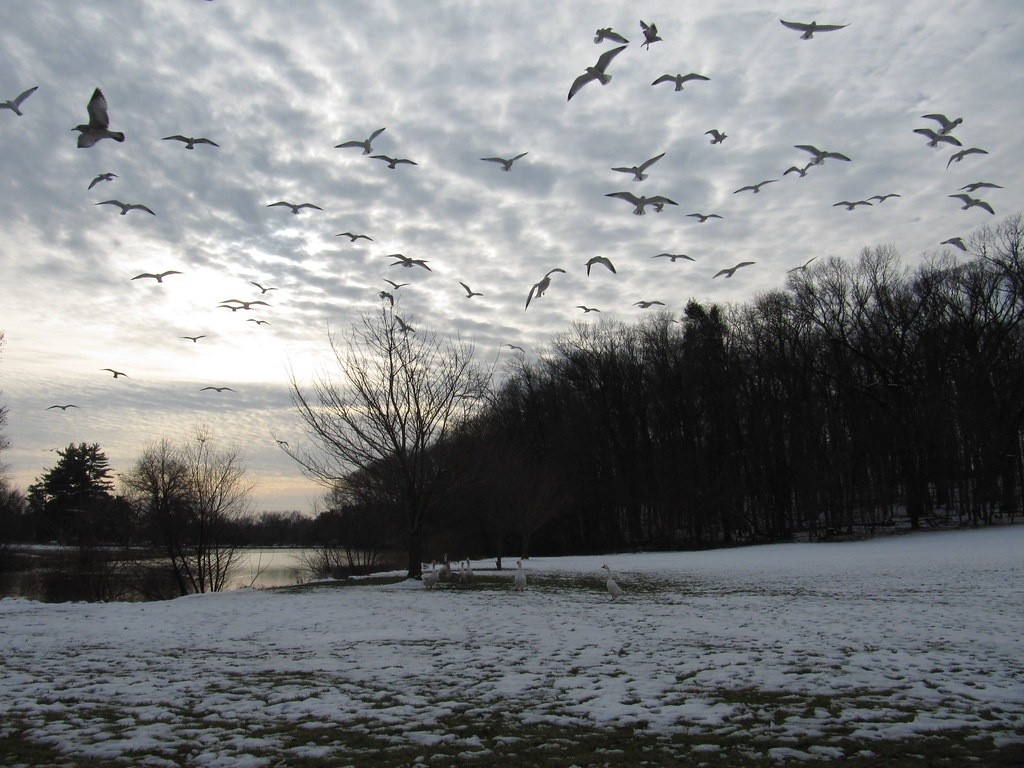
[{"x1": 0, "y1": 18, "x2": 1006, "y2": 477}]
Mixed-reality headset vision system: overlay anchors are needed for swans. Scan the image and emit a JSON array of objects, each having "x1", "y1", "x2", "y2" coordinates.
[{"x1": 421, "y1": 553, "x2": 623, "y2": 600}]
